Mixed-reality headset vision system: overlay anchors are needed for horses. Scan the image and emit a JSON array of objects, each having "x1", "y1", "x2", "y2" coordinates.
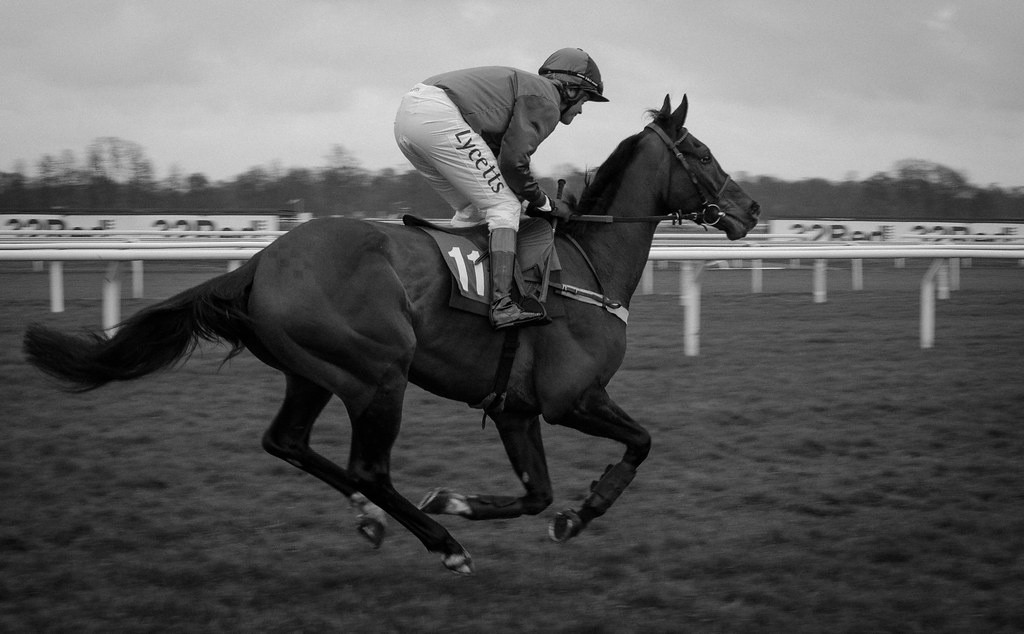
[{"x1": 21, "y1": 93, "x2": 762, "y2": 576}]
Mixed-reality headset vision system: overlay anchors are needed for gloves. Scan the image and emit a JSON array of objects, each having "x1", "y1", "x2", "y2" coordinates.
[{"x1": 540, "y1": 195, "x2": 575, "y2": 221}]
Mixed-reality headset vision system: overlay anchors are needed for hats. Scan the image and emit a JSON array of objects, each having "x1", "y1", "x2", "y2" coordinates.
[{"x1": 542, "y1": 44, "x2": 610, "y2": 102}]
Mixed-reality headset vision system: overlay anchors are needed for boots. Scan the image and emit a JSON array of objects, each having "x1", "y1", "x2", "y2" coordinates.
[{"x1": 486, "y1": 231, "x2": 550, "y2": 329}]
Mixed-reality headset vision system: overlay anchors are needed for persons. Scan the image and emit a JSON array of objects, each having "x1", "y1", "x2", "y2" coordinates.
[{"x1": 395, "y1": 48, "x2": 611, "y2": 333}]
